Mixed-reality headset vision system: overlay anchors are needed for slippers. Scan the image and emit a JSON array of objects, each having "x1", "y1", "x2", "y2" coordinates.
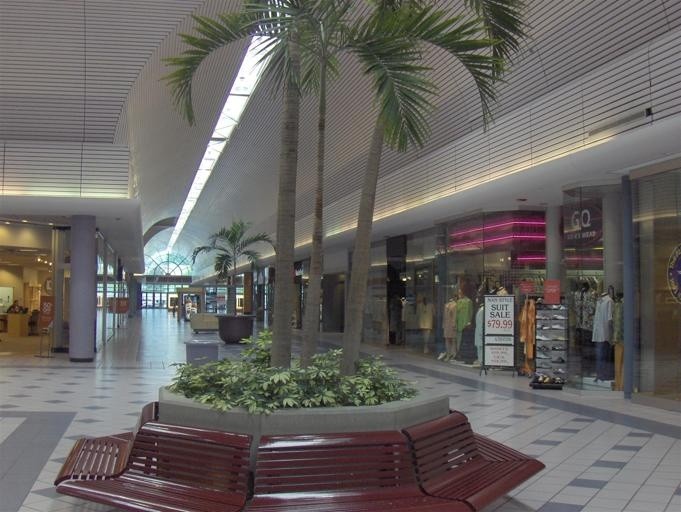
[{"x1": 551, "y1": 356, "x2": 565, "y2": 363}]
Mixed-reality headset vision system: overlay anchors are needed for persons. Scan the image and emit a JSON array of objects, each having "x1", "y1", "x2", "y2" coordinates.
[
  {"x1": 7, "y1": 300, "x2": 23, "y2": 313},
  {"x1": 611, "y1": 293, "x2": 626, "y2": 389},
  {"x1": 590, "y1": 291, "x2": 615, "y2": 381},
  {"x1": 400, "y1": 286, "x2": 510, "y2": 365}
]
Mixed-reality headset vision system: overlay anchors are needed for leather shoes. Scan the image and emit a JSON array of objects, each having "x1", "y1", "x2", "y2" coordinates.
[{"x1": 536, "y1": 334, "x2": 551, "y2": 341}]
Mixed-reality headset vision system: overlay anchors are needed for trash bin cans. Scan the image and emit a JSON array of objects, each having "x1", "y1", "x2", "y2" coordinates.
[{"x1": 183, "y1": 339, "x2": 221, "y2": 369}]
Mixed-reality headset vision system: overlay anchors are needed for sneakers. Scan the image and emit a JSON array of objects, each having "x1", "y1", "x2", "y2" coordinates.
[
  {"x1": 551, "y1": 324, "x2": 567, "y2": 331},
  {"x1": 551, "y1": 314, "x2": 568, "y2": 320},
  {"x1": 536, "y1": 353, "x2": 552, "y2": 359},
  {"x1": 552, "y1": 303, "x2": 568, "y2": 310},
  {"x1": 536, "y1": 345, "x2": 549, "y2": 352},
  {"x1": 437, "y1": 351, "x2": 452, "y2": 362},
  {"x1": 552, "y1": 344, "x2": 567, "y2": 351},
  {"x1": 536, "y1": 312, "x2": 550, "y2": 320},
  {"x1": 551, "y1": 336, "x2": 568, "y2": 340},
  {"x1": 532, "y1": 364, "x2": 568, "y2": 384},
  {"x1": 536, "y1": 323, "x2": 550, "y2": 330}
]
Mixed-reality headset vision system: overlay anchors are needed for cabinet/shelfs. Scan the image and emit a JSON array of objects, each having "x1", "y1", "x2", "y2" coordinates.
[{"x1": 529, "y1": 303, "x2": 569, "y2": 390}]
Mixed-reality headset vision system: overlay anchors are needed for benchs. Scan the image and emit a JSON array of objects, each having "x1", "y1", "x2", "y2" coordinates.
[
  {"x1": 252, "y1": 432, "x2": 472, "y2": 512},
  {"x1": 52, "y1": 400, "x2": 155, "y2": 483},
  {"x1": 56, "y1": 422, "x2": 253, "y2": 511},
  {"x1": 450, "y1": 408, "x2": 534, "y2": 461},
  {"x1": 404, "y1": 411, "x2": 544, "y2": 506}
]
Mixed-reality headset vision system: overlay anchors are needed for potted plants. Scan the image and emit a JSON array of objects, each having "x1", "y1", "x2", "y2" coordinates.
[{"x1": 193, "y1": 218, "x2": 277, "y2": 344}]
